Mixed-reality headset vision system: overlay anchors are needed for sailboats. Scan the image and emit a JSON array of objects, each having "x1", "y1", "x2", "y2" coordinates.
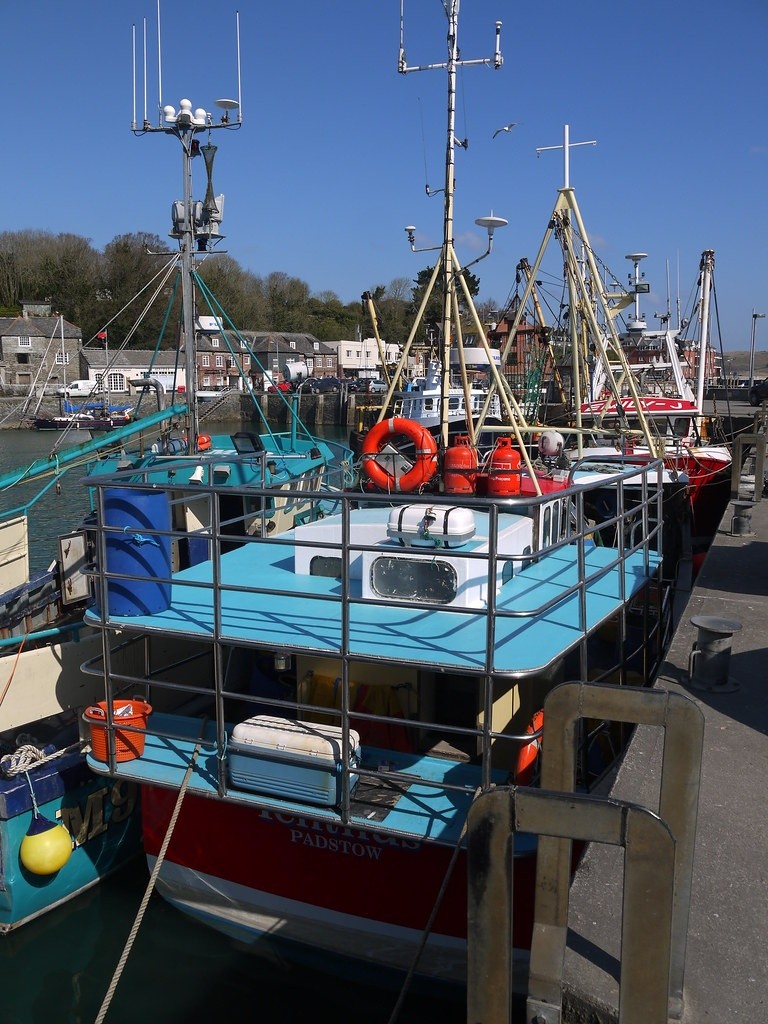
[{"x1": 13, "y1": 313, "x2": 143, "y2": 431}]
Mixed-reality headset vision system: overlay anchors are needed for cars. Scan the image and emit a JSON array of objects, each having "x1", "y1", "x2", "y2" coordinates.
[
  {"x1": 748, "y1": 377, "x2": 768, "y2": 409},
  {"x1": 302, "y1": 378, "x2": 342, "y2": 394},
  {"x1": 361, "y1": 379, "x2": 388, "y2": 394},
  {"x1": 267, "y1": 380, "x2": 292, "y2": 394}
]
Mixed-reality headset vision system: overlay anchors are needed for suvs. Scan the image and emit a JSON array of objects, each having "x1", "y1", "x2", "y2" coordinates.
[
  {"x1": 408, "y1": 377, "x2": 437, "y2": 391},
  {"x1": 345, "y1": 378, "x2": 376, "y2": 393}
]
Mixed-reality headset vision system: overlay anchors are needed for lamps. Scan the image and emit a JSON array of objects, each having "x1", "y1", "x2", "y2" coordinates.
[{"x1": 229, "y1": 431, "x2": 265, "y2": 456}]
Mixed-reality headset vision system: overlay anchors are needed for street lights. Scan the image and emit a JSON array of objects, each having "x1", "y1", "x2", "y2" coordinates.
[{"x1": 749, "y1": 307, "x2": 768, "y2": 392}]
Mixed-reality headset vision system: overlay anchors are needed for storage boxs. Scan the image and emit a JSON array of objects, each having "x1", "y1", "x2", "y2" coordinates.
[{"x1": 227, "y1": 715, "x2": 359, "y2": 805}]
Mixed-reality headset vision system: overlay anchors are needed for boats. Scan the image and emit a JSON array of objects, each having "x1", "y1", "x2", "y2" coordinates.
[{"x1": 0, "y1": 2, "x2": 740, "y2": 1007}]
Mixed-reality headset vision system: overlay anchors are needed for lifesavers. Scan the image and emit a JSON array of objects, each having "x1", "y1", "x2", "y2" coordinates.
[
  {"x1": 181, "y1": 433, "x2": 212, "y2": 451},
  {"x1": 125, "y1": 416, "x2": 128, "y2": 420},
  {"x1": 513, "y1": 707, "x2": 545, "y2": 786},
  {"x1": 361, "y1": 416, "x2": 438, "y2": 494}
]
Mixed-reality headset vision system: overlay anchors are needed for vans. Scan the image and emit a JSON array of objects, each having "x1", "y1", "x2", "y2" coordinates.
[{"x1": 56, "y1": 379, "x2": 99, "y2": 398}]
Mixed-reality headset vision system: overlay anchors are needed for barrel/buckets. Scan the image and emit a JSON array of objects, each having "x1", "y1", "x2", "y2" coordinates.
[{"x1": 84, "y1": 700, "x2": 152, "y2": 763}]
